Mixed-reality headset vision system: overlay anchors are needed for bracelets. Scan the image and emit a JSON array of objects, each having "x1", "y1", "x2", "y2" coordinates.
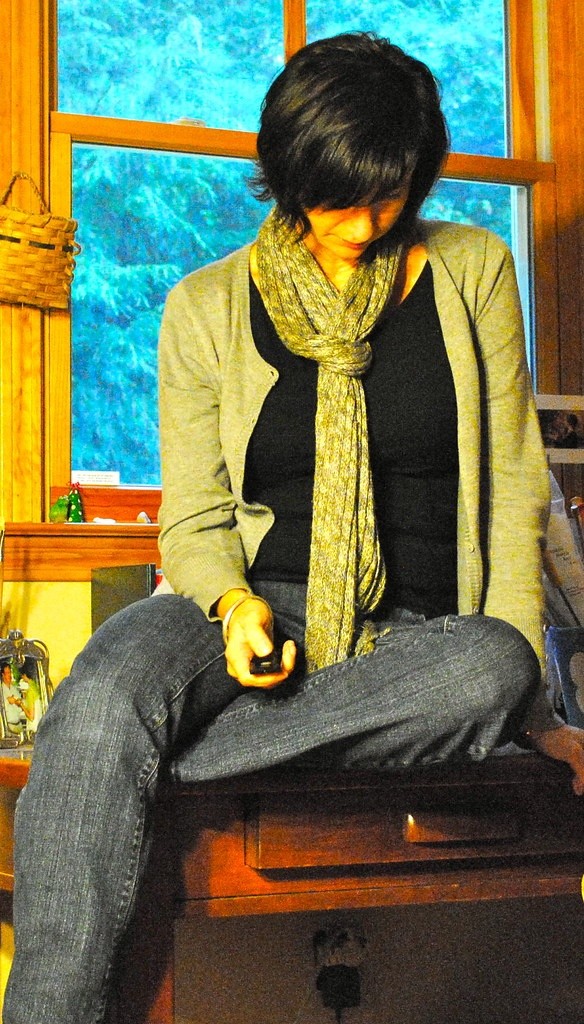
[{"x1": 221, "y1": 594, "x2": 273, "y2": 644}]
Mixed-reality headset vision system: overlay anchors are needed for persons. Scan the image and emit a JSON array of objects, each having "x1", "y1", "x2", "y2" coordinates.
[
  {"x1": 1, "y1": 663, "x2": 24, "y2": 734},
  {"x1": 0, "y1": 32, "x2": 584, "y2": 1024},
  {"x1": 15, "y1": 664, "x2": 43, "y2": 737}
]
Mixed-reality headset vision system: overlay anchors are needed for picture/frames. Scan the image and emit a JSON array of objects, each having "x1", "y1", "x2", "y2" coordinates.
[
  {"x1": 535, "y1": 393, "x2": 584, "y2": 464},
  {"x1": 0, "y1": 628, "x2": 54, "y2": 751}
]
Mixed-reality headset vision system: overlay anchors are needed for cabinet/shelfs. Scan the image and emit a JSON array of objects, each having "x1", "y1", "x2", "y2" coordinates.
[{"x1": 0, "y1": 743, "x2": 584, "y2": 1024}]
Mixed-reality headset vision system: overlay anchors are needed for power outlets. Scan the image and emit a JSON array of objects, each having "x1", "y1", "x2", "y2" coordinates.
[{"x1": 312, "y1": 926, "x2": 366, "y2": 1011}]
